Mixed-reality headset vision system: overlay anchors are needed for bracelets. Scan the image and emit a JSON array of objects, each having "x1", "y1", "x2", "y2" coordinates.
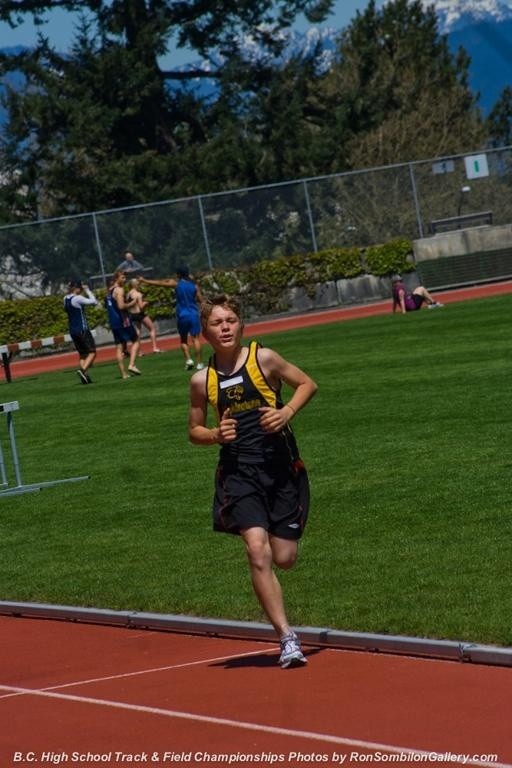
[{"x1": 285, "y1": 404, "x2": 297, "y2": 414}]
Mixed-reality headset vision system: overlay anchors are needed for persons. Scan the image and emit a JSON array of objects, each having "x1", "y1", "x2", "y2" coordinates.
[
  {"x1": 139, "y1": 265, "x2": 206, "y2": 372},
  {"x1": 104, "y1": 271, "x2": 145, "y2": 380},
  {"x1": 62, "y1": 278, "x2": 100, "y2": 385},
  {"x1": 389, "y1": 274, "x2": 442, "y2": 315},
  {"x1": 117, "y1": 252, "x2": 143, "y2": 280},
  {"x1": 185, "y1": 292, "x2": 322, "y2": 671},
  {"x1": 126, "y1": 277, "x2": 163, "y2": 358}
]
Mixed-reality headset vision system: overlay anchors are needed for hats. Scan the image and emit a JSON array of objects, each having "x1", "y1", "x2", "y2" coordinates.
[
  {"x1": 69, "y1": 279, "x2": 85, "y2": 288},
  {"x1": 391, "y1": 275, "x2": 403, "y2": 282},
  {"x1": 174, "y1": 266, "x2": 189, "y2": 277}
]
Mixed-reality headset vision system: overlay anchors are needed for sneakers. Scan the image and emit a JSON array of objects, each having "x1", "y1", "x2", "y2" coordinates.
[
  {"x1": 197, "y1": 363, "x2": 204, "y2": 370},
  {"x1": 427, "y1": 301, "x2": 445, "y2": 309},
  {"x1": 77, "y1": 367, "x2": 91, "y2": 384},
  {"x1": 121, "y1": 347, "x2": 164, "y2": 358},
  {"x1": 279, "y1": 633, "x2": 308, "y2": 669},
  {"x1": 123, "y1": 375, "x2": 131, "y2": 380},
  {"x1": 128, "y1": 365, "x2": 141, "y2": 376},
  {"x1": 185, "y1": 359, "x2": 195, "y2": 371},
  {"x1": 85, "y1": 374, "x2": 96, "y2": 384}
]
